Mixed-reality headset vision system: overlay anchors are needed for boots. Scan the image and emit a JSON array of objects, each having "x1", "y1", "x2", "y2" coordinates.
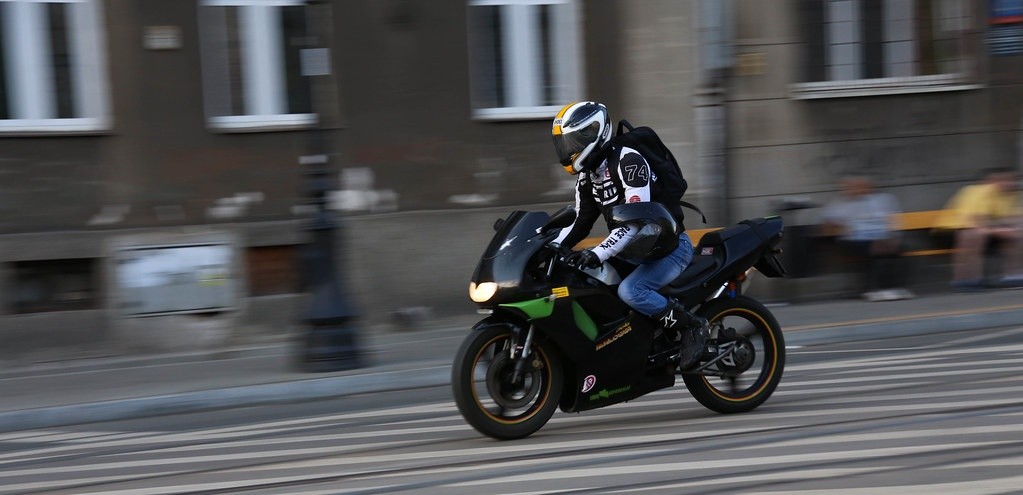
[{"x1": 651, "y1": 296, "x2": 712, "y2": 371}]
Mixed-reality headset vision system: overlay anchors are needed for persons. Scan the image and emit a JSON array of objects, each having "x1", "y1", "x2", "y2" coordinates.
[
  {"x1": 823, "y1": 174, "x2": 917, "y2": 302},
  {"x1": 930, "y1": 164, "x2": 1023, "y2": 291},
  {"x1": 546, "y1": 101, "x2": 710, "y2": 367}
]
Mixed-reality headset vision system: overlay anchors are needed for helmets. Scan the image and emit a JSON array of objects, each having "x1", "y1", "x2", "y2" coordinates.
[
  {"x1": 606, "y1": 202, "x2": 679, "y2": 265},
  {"x1": 552, "y1": 101, "x2": 613, "y2": 175}
]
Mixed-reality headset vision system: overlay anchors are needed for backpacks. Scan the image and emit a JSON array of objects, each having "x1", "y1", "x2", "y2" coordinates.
[{"x1": 586, "y1": 119, "x2": 707, "y2": 224}]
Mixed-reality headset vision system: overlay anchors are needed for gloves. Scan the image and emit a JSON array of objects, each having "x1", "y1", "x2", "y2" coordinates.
[{"x1": 564, "y1": 249, "x2": 604, "y2": 273}]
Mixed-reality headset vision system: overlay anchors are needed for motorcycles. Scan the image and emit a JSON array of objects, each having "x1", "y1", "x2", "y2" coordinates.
[{"x1": 447, "y1": 201, "x2": 791, "y2": 445}]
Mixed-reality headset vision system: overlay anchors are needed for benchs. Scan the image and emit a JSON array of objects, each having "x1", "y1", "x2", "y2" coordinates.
[{"x1": 887, "y1": 206, "x2": 967, "y2": 257}]
